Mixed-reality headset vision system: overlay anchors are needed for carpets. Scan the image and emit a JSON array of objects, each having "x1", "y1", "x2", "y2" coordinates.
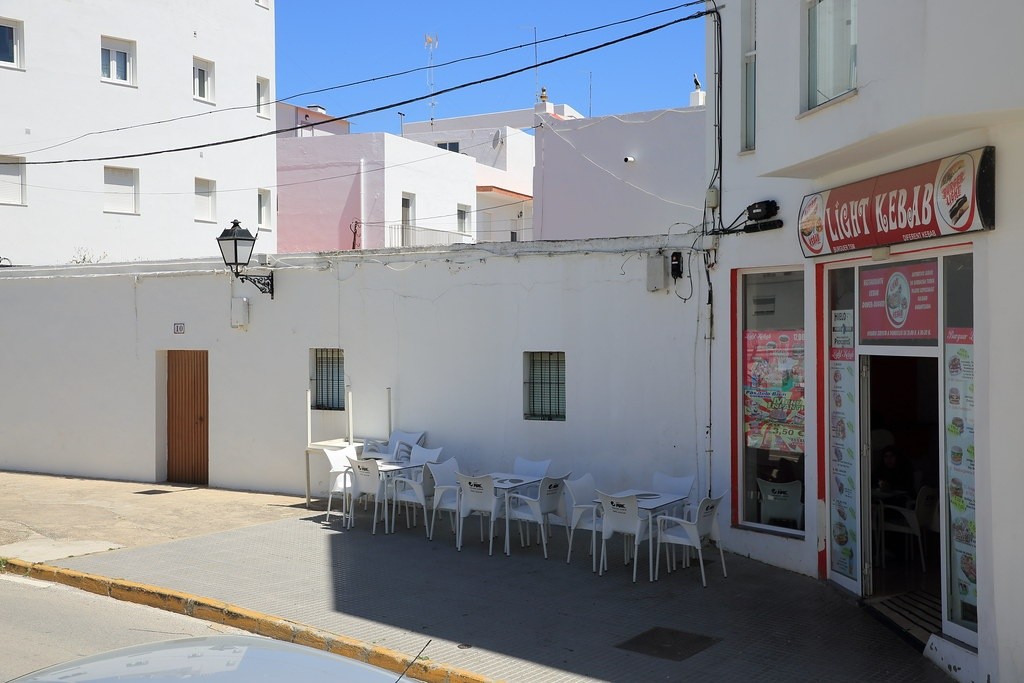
[{"x1": 863, "y1": 582, "x2": 942, "y2": 646}]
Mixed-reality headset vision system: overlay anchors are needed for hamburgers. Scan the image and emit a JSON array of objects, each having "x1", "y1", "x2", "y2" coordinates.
[
  {"x1": 939, "y1": 159, "x2": 970, "y2": 225},
  {"x1": 948, "y1": 354, "x2": 965, "y2": 497},
  {"x1": 801, "y1": 198, "x2": 819, "y2": 237}
]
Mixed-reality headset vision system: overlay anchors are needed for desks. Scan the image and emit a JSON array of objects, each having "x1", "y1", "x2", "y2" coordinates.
[
  {"x1": 591, "y1": 487, "x2": 688, "y2": 583},
  {"x1": 343, "y1": 458, "x2": 424, "y2": 535},
  {"x1": 454, "y1": 471, "x2": 544, "y2": 553},
  {"x1": 305, "y1": 385, "x2": 392, "y2": 508}
]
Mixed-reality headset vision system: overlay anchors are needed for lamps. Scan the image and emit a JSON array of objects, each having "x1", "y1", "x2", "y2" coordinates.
[{"x1": 219, "y1": 218, "x2": 274, "y2": 298}]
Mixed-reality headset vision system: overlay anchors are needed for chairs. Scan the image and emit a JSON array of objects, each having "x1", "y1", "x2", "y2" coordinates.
[
  {"x1": 323, "y1": 432, "x2": 730, "y2": 588},
  {"x1": 872, "y1": 472, "x2": 939, "y2": 574},
  {"x1": 754, "y1": 477, "x2": 805, "y2": 532}
]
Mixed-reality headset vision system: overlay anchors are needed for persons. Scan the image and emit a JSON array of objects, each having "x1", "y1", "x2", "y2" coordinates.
[{"x1": 873, "y1": 446, "x2": 914, "y2": 559}]
